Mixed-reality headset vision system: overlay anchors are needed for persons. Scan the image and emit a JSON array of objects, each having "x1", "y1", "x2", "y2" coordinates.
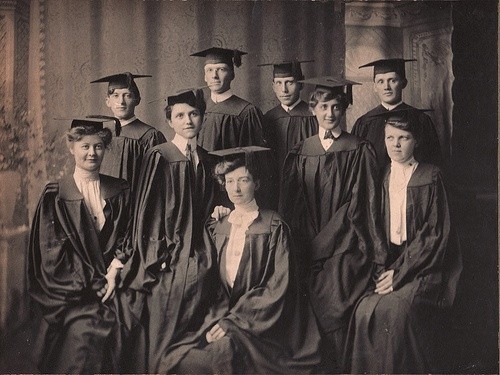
[
  {"x1": 260, "y1": 60, "x2": 319, "y2": 171},
  {"x1": 351, "y1": 60, "x2": 441, "y2": 176},
  {"x1": 277, "y1": 77, "x2": 381, "y2": 375},
  {"x1": 119, "y1": 85, "x2": 235, "y2": 375},
  {"x1": 23, "y1": 114, "x2": 136, "y2": 375},
  {"x1": 336, "y1": 109, "x2": 474, "y2": 375},
  {"x1": 169, "y1": 146, "x2": 322, "y2": 375},
  {"x1": 190, "y1": 46, "x2": 266, "y2": 150},
  {"x1": 90, "y1": 72, "x2": 168, "y2": 179}
]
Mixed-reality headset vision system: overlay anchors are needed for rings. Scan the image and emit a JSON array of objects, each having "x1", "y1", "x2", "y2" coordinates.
[{"x1": 389, "y1": 287, "x2": 393, "y2": 292}]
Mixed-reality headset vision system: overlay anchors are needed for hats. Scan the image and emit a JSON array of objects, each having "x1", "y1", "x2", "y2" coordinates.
[
  {"x1": 89, "y1": 72, "x2": 153, "y2": 90},
  {"x1": 357, "y1": 58, "x2": 417, "y2": 78},
  {"x1": 258, "y1": 59, "x2": 316, "y2": 79},
  {"x1": 366, "y1": 109, "x2": 435, "y2": 123},
  {"x1": 146, "y1": 84, "x2": 212, "y2": 111},
  {"x1": 207, "y1": 146, "x2": 272, "y2": 172},
  {"x1": 295, "y1": 75, "x2": 363, "y2": 106},
  {"x1": 52, "y1": 114, "x2": 123, "y2": 138},
  {"x1": 188, "y1": 47, "x2": 248, "y2": 68}
]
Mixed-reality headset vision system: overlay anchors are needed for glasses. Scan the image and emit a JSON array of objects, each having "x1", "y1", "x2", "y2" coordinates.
[{"x1": 221, "y1": 177, "x2": 256, "y2": 186}]
